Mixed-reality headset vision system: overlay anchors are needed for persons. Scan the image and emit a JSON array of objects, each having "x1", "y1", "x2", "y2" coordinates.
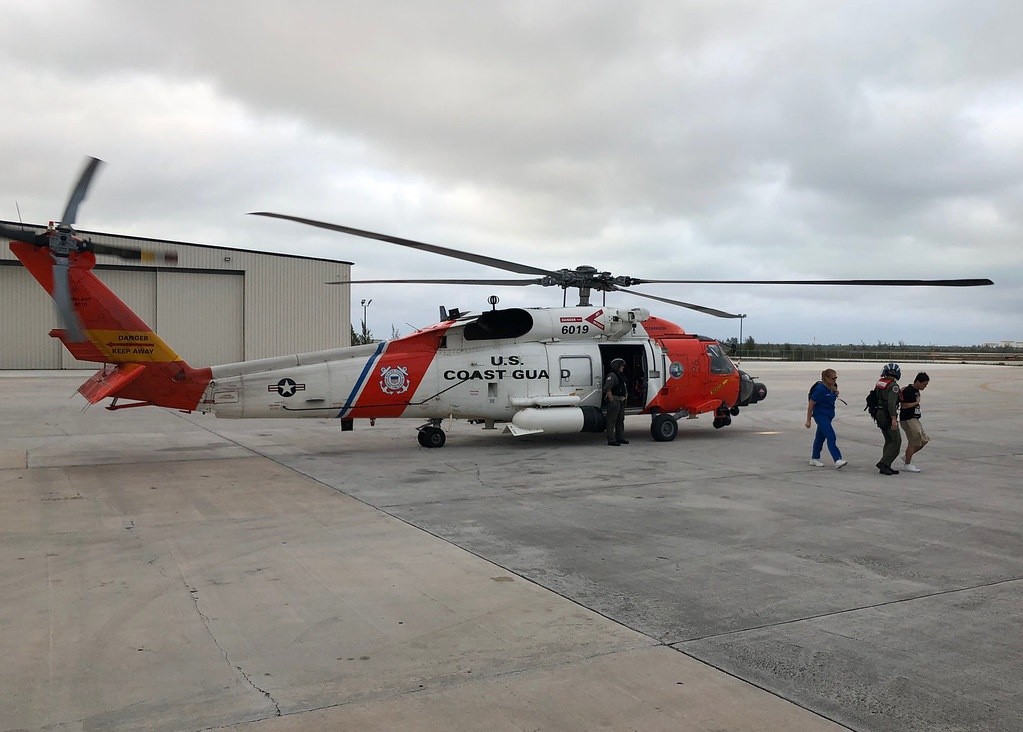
[
  {"x1": 874, "y1": 363, "x2": 902, "y2": 475},
  {"x1": 805, "y1": 369, "x2": 847, "y2": 469},
  {"x1": 899, "y1": 372, "x2": 930, "y2": 473},
  {"x1": 604, "y1": 358, "x2": 629, "y2": 446}
]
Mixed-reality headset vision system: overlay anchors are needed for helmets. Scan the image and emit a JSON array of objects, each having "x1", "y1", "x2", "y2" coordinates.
[
  {"x1": 610, "y1": 358, "x2": 626, "y2": 370},
  {"x1": 879, "y1": 363, "x2": 902, "y2": 380}
]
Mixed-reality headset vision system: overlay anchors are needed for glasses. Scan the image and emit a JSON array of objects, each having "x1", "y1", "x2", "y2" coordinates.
[{"x1": 831, "y1": 377, "x2": 838, "y2": 380}]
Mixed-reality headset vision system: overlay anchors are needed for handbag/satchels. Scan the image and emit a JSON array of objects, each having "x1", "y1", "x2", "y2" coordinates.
[
  {"x1": 863, "y1": 388, "x2": 878, "y2": 422},
  {"x1": 807, "y1": 381, "x2": 823, "y2": 417}
]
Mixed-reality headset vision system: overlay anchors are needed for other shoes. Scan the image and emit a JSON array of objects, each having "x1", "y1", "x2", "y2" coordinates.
[
  {"x1": 607, "y1": 441, "x2": 621, "y2": 446},
  {"x1": 876, "y1": 461, "x2": 892, "y2": 475},
  {"x1": 616, "y1": 439, "x2": 629, "y2": 444},
  {"x1": 879, "y1": 467, "x2": 899, "y2": 474}
]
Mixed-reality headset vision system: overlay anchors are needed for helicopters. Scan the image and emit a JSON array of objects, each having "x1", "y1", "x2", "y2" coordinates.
[{"x1": 0, "y1": 152, "x2": 993, "y2": 449}]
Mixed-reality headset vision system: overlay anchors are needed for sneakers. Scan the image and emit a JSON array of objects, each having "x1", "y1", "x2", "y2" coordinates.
[
  {"x1": 808, "y1": 458, "x2": 824, "y2": 467},
  {"x1": 903, "y1": 464, "x2": 921, "y2": 472},
  {"x1": 833, "y1": 460, "x2": 848, "y2": 470}
]
